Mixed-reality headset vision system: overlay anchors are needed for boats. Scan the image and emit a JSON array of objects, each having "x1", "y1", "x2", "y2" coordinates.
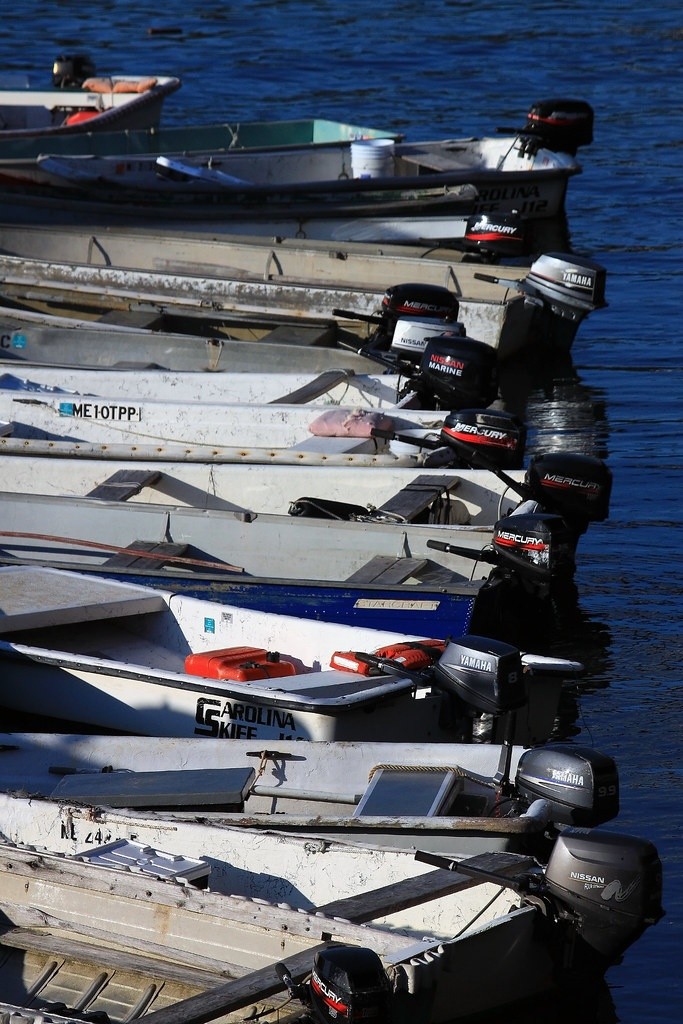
[
  {"x1": 0, "y1": 491, "x2": 576, "y2": 620},
  {"x1": 1, "y1": 785, "x2": 665, "y2": 1023},
  {"x1": 4, "y1": 314, "x2": 502, "y2": 436},
  {"x1": 4, "y1": 282, "x2": 464, "y2": 362},
  {"x1": 0, "y1": 228, "x2": 612, "y2": 385},
  {"x1": 1, "y1": 169, "x2": 547, "y2": 272},
  {"x1": 0, "y1": 436, "x2": 616, "y2": 583},
  {"x1": 1, "y1": 52, "x2": 183, "y2": 140},
  {"x1": 0, "y1": 733, "x2": 619, "y2": 870},
  {"x1": 0, "y1": 382, "x2": 519, "y2": 489},
  {"x1": 0, "y1": 565, "x2": 581, "y2": 758},
  {"x1": 1, "y1": 97, "x2": 594, "y2": 243},
  {"x1": 0, "y1": 292, "x2": 467, "y2": 390}
]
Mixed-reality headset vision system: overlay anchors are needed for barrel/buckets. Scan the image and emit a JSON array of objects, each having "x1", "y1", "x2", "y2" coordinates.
[{"x1": 349, "y1": 138, "x2": 396, "y2": 180}]
